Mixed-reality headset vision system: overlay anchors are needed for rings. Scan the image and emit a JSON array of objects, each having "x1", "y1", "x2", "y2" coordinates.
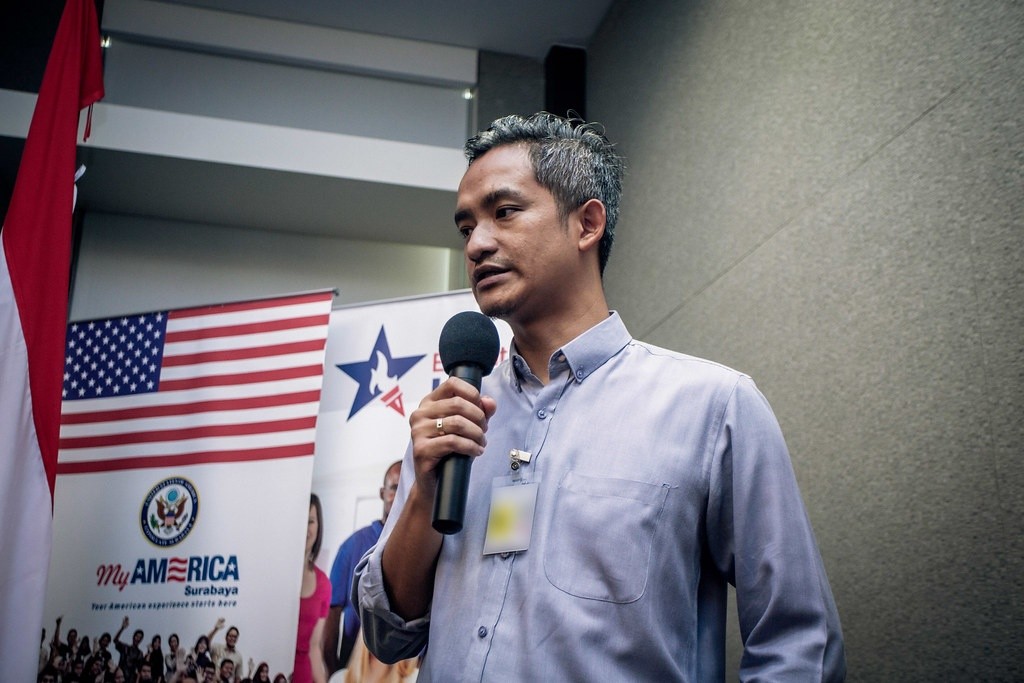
[{"x1": 437, "y1": 418, "x2": 445, "y2": 436}]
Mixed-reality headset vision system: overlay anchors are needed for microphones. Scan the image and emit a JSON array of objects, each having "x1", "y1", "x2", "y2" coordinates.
[{"x1": 433, "y1": 311, "x2": 500, "y2": 535}]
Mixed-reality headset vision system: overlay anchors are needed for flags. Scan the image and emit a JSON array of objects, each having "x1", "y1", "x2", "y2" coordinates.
[{"x1": 0, "y1": 1, "x2": 105, "y2": 683}]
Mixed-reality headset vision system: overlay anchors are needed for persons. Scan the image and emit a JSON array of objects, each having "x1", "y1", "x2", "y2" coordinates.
[
  {"x1": 292, "y1": 493, "x2": 334, "y2": 683},
  {"x1": 38, "y1": 615, "x2": 289, "y2": 683},
  {"x1": 327, "y1": 632, "x2": 420, "y2": 683},
  {"x1": 321, "y1": 460, "x2": 403, "y2": 673},
  {"x1": 347, "y1": 111, "x2": 847, "y2": 683}
]
